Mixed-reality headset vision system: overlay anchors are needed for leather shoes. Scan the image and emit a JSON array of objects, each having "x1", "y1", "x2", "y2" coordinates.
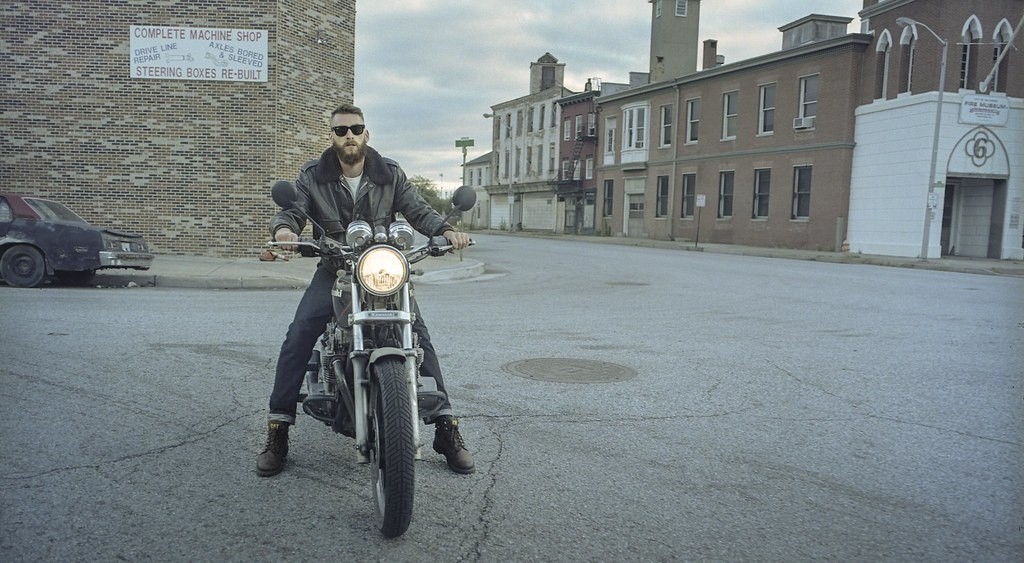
[
  {"x1": 432, "y1": 415, "x2": 475, "y2": 474},
  {"x1": 257, "y1": 420, "x2": 288, "y2": 477}
]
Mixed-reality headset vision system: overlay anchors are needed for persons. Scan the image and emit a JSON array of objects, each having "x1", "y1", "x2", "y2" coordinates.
[{"x1": 254, "y1": 103, "x2": 477, "y2": 477}]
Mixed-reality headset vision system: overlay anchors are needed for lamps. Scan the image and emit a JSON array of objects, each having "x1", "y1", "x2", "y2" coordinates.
[{"x1": 317, "y1": 28, "x2": 328, "y2": 44}]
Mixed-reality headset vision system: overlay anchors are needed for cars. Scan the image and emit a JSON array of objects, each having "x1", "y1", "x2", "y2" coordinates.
[{"x1": 0, "y1": 191, "x2": 154, "y2": 290}]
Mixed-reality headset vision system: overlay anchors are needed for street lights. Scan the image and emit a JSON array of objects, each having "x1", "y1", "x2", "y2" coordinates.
[
  {"x1": 483, "y1": 113, "x2": 512, "y2": 233},
  {"x1": 895, "y1": 16, "x2": 949, "y2": 257}
]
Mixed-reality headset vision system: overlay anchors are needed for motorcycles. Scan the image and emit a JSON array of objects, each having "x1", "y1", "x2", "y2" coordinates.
[{"x1": 263, "y1": 178, "x2": 479, "y2": 535}]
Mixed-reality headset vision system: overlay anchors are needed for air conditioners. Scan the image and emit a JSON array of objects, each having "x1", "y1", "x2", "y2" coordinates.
[{"x1": 793, "y1": 117, "x2": 813, "y2": 128}]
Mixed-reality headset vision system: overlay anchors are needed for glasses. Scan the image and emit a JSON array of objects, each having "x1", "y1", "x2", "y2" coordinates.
[{"x1": 331, "y1": 124, "x2": 365, "y2": 137}]
